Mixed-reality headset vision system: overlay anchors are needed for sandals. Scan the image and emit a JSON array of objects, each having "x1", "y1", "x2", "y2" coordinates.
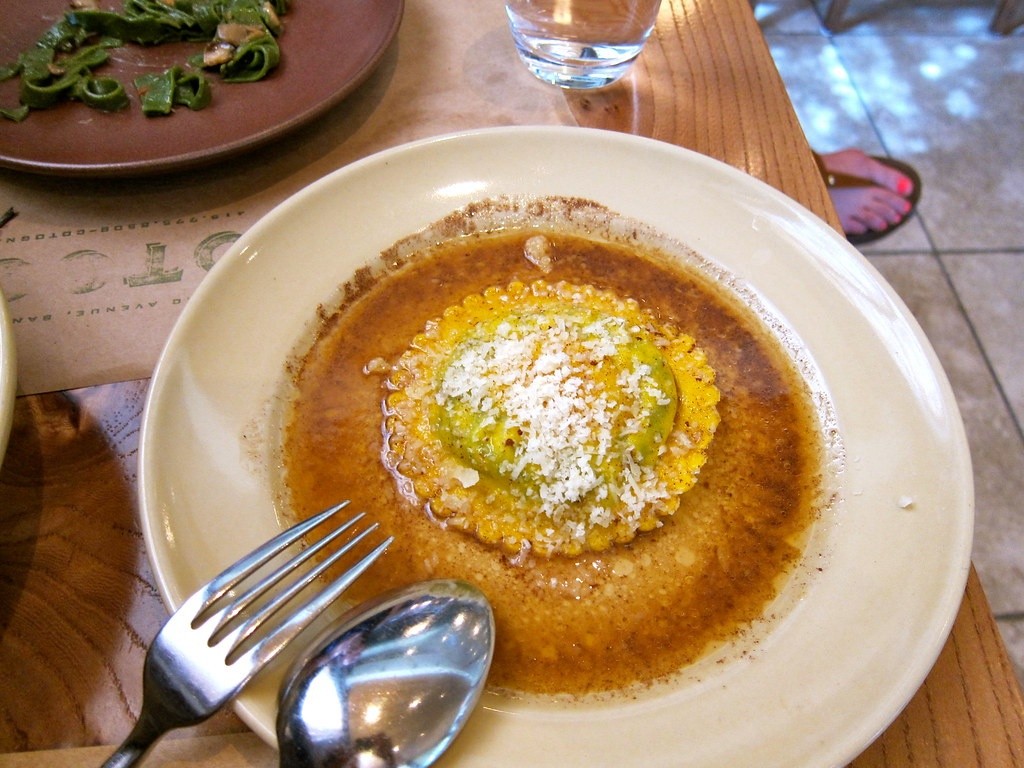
[{"x1": 813, "y1": 147, "x2": 921, "y2": 245}]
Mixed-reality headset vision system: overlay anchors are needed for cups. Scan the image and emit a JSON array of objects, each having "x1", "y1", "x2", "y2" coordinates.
[{"x1": 504, "y1": 0, "x2": 663, "y2": 90}]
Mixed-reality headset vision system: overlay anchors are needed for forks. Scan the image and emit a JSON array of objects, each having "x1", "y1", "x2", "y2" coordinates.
[{"x1": 101, "y1": 499, "x2": 394, "y2": 768}]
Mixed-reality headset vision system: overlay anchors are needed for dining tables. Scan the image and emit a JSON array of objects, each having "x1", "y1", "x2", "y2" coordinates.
[{"x1": 0, "y1": 0, "x2": 1024, "y2": 768}]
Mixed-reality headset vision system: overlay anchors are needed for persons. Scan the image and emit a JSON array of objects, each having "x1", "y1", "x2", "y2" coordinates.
[{"x1": 814, "y1": 149, "x2": 920, "y2": 241}]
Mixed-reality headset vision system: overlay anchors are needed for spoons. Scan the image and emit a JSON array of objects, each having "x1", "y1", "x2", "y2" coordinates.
[{"x1": 274, "y1": 576, "x2": 496, "y2": 768}]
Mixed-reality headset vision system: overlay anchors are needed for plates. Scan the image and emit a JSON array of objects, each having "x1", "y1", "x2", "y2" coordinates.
[
  {"x1": 136, "y1": 126, "x2": 977, "y2": 768},
  {"x1": 0, "y1": 0, "x2": 407, "y2": 175}
]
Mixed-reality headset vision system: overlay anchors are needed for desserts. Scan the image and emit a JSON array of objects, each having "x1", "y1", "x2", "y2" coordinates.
[{"x1": 383, "y1": 279, "x2": 720, "y2": 556}]
